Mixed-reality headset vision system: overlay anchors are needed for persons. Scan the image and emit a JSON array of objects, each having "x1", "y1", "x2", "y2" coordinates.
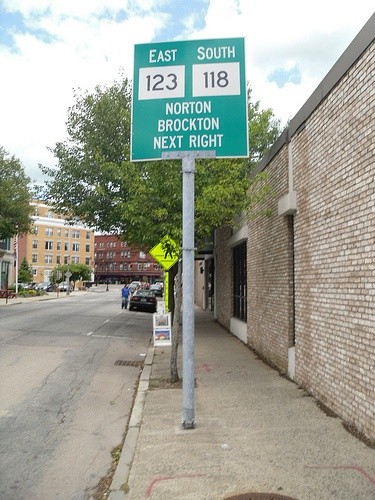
[{"x1": 121, "y1": 283, "x2": 130, "y2": 309}]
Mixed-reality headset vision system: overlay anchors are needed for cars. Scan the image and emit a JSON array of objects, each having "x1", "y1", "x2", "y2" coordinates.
[
  {"x1": 129, "y1": 281, "x2": 162, "y2": 296},
  {"x1": 129, "y1": 289, "x2": 157, "y2": 312},
  {"x1": 19, "y1": 282, "x2": 74, "y2": 292}
]
